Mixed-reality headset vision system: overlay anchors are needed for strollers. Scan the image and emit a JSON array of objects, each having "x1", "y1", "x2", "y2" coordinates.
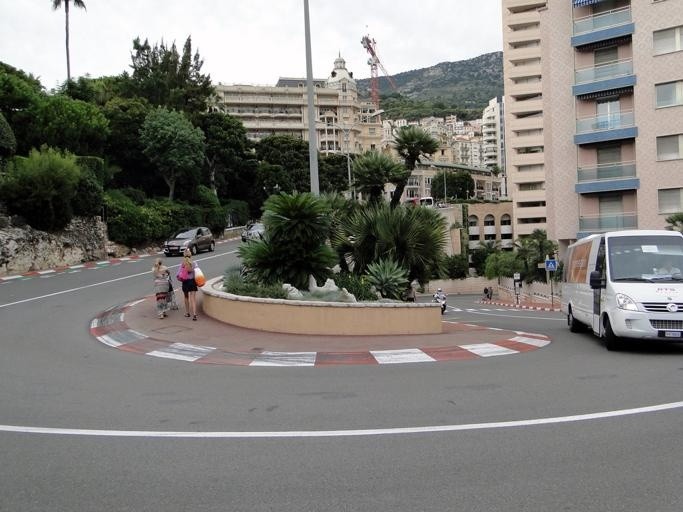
[{"x1": 165, "y1": 277, "x2": 178, "y2": 312}]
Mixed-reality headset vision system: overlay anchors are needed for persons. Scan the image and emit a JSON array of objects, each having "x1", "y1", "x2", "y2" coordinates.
[
  {"x1": 484, "y1": 287, "x2": 489, "y2": 298},
  {"x1": 178, "y1": 248, "x2": 199, "y2": 320},
  {"x1": 433, "y1": 288, "x2": 446, "y2": 302},
  {"x1": 152, "y1": 258, "x2": 178, "y2": 318},
  {"x1": 488, "y1": 286, "x2": 493, "y2": 299},
  {"x1": 403, "y1": 286, "x2": 417, "y2": 302}
]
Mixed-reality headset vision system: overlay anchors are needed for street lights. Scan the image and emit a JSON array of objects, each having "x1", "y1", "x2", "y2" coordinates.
[
  {"x1": 313, "y1": 109, "x2": 387, "y2": 200},
  {"x1": 465, "y1": 189, "x2": 469, "y2": 199},
  {"x1": 429, "y1": 163, "x2": 446, "y2": 205}
]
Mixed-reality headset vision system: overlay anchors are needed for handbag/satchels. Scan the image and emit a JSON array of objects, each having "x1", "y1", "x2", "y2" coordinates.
[
  {"x1": 176, "y1": 262, "x2": 190, "y2": 282},
  {"x1": 193, "y1": 261, "x2": 206, "y2": 287}
]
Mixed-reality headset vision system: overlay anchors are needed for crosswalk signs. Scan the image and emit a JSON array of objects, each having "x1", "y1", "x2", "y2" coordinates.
[{"x1": 543, "y1": 260, "x2": 557, "y2": 272}]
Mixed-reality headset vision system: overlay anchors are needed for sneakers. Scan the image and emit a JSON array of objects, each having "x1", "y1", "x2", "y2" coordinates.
[
  {"x1": 193, "y1": 315, "x2": 198, "y2": 320},
  {"x1": 183, "y1": 312, "x2": 190, "y2": 317},
  {"x1": 158, "y1": 311, "x2": 168, "y2": 319}
]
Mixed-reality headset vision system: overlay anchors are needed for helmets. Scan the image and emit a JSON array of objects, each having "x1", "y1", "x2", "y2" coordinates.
[{"x1": 437, "y1": 288, "x2": 442, "y2": 291}]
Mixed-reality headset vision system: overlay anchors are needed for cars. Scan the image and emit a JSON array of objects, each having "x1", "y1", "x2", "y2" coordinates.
[
  {"x1": 403, "y1": 195, "x2": 448, "y2": 211},
  {"x1": 240, "y1": 222, "x2": 265, "y2": 243}
]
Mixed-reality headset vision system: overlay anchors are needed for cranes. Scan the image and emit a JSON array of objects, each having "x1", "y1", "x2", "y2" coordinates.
[{"x1": 359, "y1": 31, "x2": 396, "y2": 102}]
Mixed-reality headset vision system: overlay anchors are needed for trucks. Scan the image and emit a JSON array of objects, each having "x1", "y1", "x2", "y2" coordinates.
[{"x1": 558, "y1": 227, "x2": 682, "y2": 351}]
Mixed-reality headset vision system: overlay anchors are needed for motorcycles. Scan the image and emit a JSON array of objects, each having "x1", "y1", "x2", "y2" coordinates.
[{"x1": 431, "y1": 293, "x2": 447, "y2": 315}]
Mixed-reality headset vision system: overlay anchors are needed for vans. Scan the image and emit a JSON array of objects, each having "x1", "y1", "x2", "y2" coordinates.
[{"x1": 162, "y1": 225, "x2": 215, "y2": 257}]
